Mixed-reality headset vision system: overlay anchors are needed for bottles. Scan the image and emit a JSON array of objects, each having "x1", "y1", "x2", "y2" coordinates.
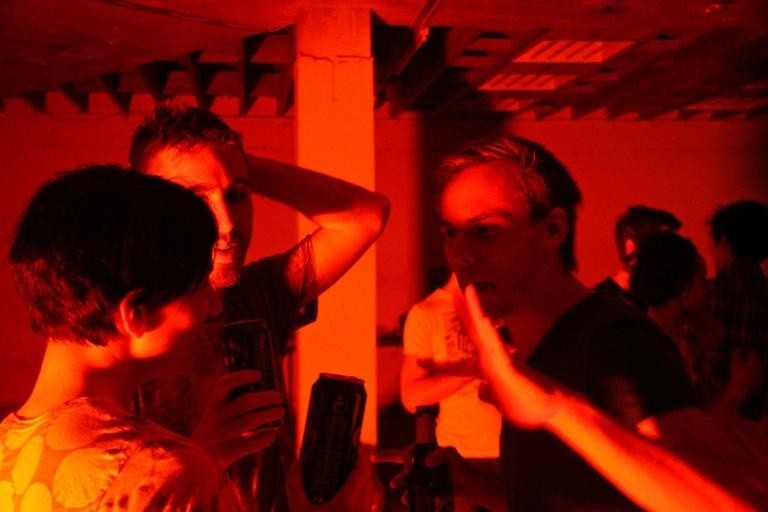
[{"x1": 408, "y1": 405, "x2": 453, "y2": 512}]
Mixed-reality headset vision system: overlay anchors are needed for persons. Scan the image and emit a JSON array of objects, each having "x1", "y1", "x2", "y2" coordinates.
[
  {"x1": 614, "y1": 198, "x2": 766, "y2": 422},
  {"x1": 391, "y1": 136, "x2": 756, "y2": 510},
  {"x1": 127, "y1": 107, "x2": 391, "y2": 512},
  {"x1": 398, "y1": 272, "x2": 517, "y2": 498},
  {"x1": 2, "y1": 162, "x2": 249, "y2": 511}
]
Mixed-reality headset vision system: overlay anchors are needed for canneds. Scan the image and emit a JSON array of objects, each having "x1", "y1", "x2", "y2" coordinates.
[
  {"x1": 402, "y1": 443, "x2": 454, "y2": 512},
  {"x1": 297, "y1": 375, "x2": 366, "y2": 503},
  {"x1": 219, "y1": 319, "x2": 289, "y2": 437}
]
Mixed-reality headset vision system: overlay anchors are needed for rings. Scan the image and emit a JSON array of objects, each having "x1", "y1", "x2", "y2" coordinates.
[{"x1": 239, "y1": 419, "x2": 254, "y2": 436}]
